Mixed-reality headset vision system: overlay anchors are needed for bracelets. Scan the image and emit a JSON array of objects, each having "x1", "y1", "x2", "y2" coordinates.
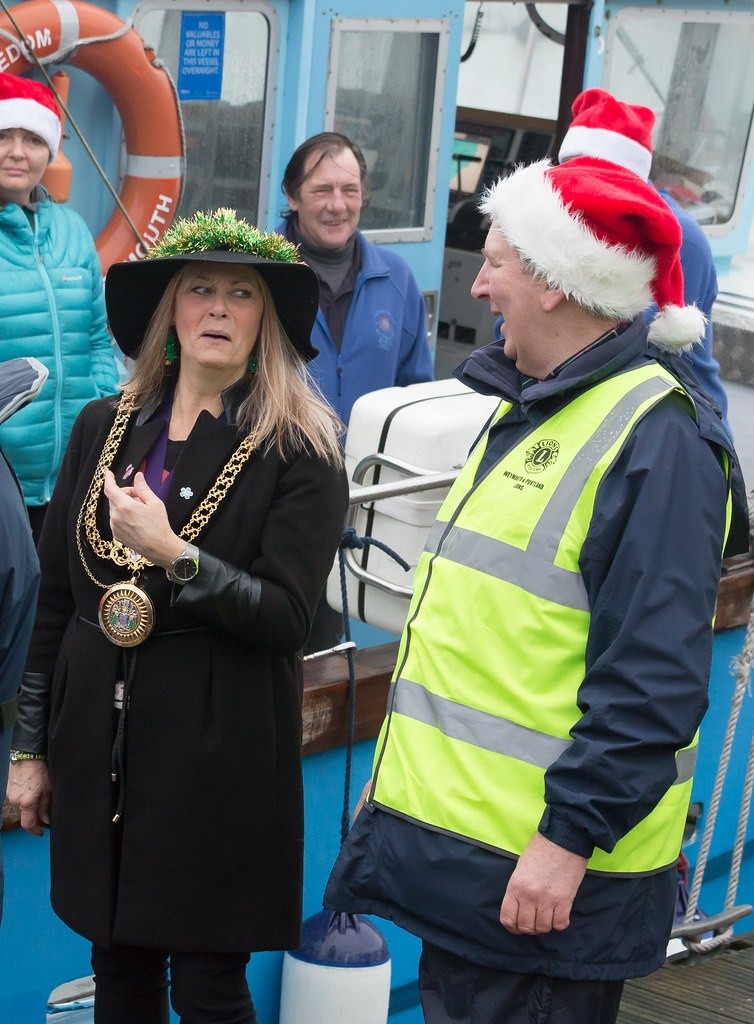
[{"x1": 9, "y1": 750, "x2": 43, "y2": 764}]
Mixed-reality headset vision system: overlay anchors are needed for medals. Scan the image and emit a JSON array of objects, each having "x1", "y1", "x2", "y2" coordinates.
[{"x1": 97, "y1": 584, "x2": 155, "y2": 646}]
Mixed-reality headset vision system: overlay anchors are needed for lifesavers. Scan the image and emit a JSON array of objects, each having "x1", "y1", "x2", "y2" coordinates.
[{"x1": 1, "y1": 0, "x2": 190, "y2": 285}]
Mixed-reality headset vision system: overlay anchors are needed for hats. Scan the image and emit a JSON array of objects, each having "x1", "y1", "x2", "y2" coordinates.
[
  {"x1": 478, "y1": 155, "x2": 708, "y2": 354},
  {"x1": 558, "y1": 87, "x2": 656, "y2": 184},
  {"x1": 0, "y1": 72, "x2": 62, "y2": 164},
  {"x1": 104, "y1": 207, "x2": 319, "y2": 364}
]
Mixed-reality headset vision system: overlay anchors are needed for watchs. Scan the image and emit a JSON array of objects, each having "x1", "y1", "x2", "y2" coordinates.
[{"x1": 166, "y1": 542, "x2": 200, "y2": 586}]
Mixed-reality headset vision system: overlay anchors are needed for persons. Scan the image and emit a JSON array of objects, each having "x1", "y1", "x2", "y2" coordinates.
[
  {"x1": 1, "y1": 67, "x2": 122, "y2": 550},
  {"x1": 0, "y1": 450, "x2": 43, "y2": 794},
  {"x1": 322, "y1": 150, "x2": 740, "y2": 1023},
  {"x1": 495, "y1": 88, "x2": 734, "y2": 439},
  {"x1": 1, "y1": 208, "x2": 352, "y2": 1024},
  {"x1": 268, "y1": 130, "x2": 436, "y2": 458}
]
